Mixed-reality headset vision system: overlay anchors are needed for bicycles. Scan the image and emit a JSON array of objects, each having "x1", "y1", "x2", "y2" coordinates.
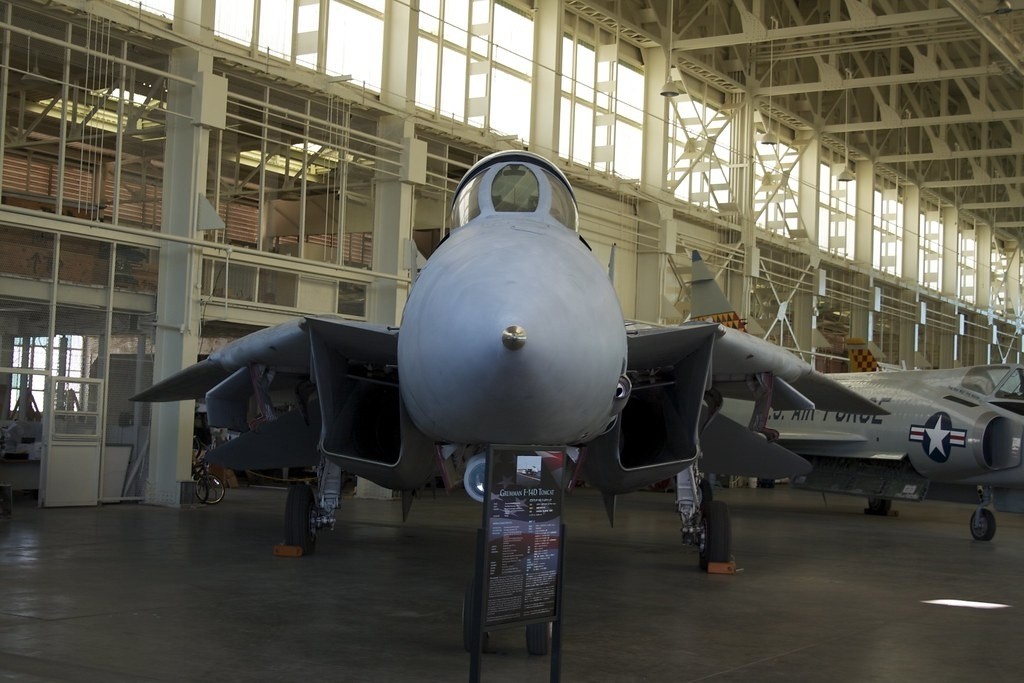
[{"x1": 190, "y1": 450, "x2": 225, "y2": 505}]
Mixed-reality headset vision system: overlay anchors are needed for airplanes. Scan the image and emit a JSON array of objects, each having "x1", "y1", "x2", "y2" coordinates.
[
  {"x1": 563, "y1": 362, "x2": 1024, "y2": 542},
  {"x1": 129, "y1": 150, "x2": 892, "y2": 652}
]
